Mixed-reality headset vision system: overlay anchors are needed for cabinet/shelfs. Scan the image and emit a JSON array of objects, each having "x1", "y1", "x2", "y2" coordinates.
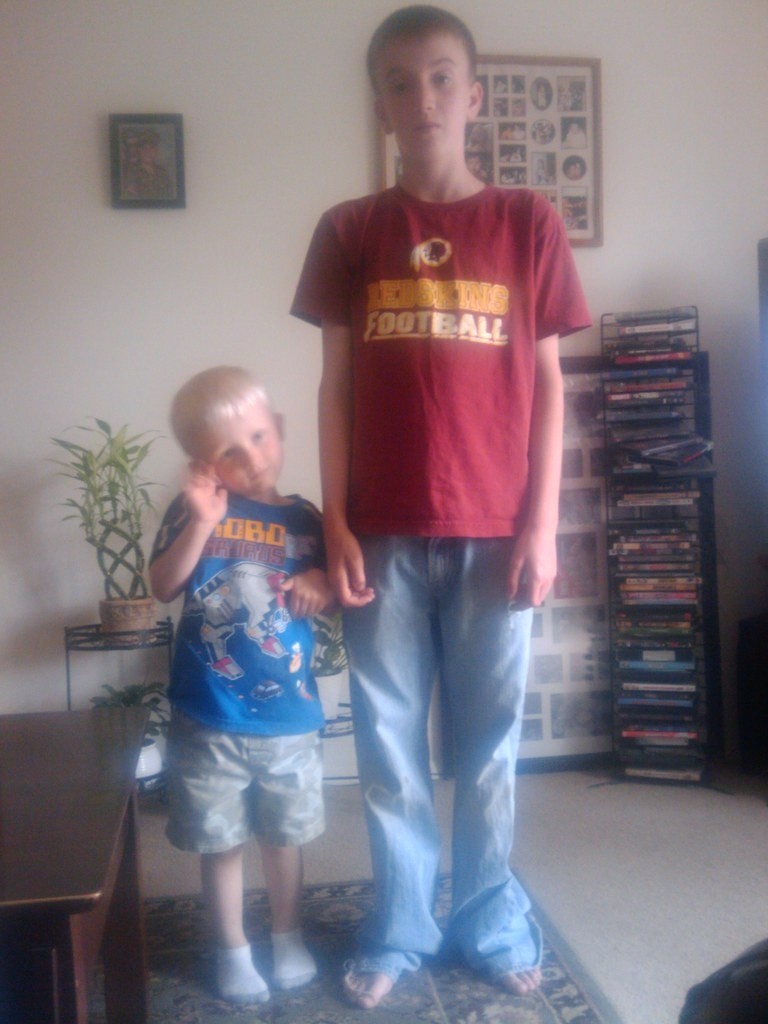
[{"x1": 600, "y1": 306, "x2": 723, "y2": 788}]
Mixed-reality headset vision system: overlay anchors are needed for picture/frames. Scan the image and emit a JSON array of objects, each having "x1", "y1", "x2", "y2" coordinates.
[
  {"x1": 109, "y1": 111, "x2": 187, "y2": 209},
  {"x1": 514, "y1": 356, "x2": 620, "y2": 774},
  {"x1": 377, "y1": 55, "x2": 602, "y2": 249}
]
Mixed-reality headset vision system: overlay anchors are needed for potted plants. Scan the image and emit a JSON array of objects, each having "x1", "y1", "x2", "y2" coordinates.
[
  {"x1": 43, "y1": 419, "x2": 157, "y2": 642},
  {"x1": 89, "y1": 673, "x2": 175, "y2": 789},
  {"x1": 309, "y1": 607, "x2": 348, "y2": 720}
]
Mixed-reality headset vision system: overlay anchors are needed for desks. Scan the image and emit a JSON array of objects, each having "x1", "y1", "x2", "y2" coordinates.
[{"x1": 0, "y1": 706, "x2": 152, "y2": 1024}]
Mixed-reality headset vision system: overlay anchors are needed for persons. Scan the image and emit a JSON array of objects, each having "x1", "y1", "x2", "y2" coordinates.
[
  {"x1": 459, "y1": 77, "x2": 589, "y2": 232},
  {"x1": 287, "y1": 9, "x2": 594, "y2": 1010},
  {"x1": 149, "y1": 367, "x2": 338, "y2": 1006}
]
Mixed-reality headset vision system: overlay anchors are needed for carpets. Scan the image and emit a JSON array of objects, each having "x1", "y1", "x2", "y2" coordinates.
[{"x1": 65, "y1": 866, "x2": 624, "y2": 1024}]
